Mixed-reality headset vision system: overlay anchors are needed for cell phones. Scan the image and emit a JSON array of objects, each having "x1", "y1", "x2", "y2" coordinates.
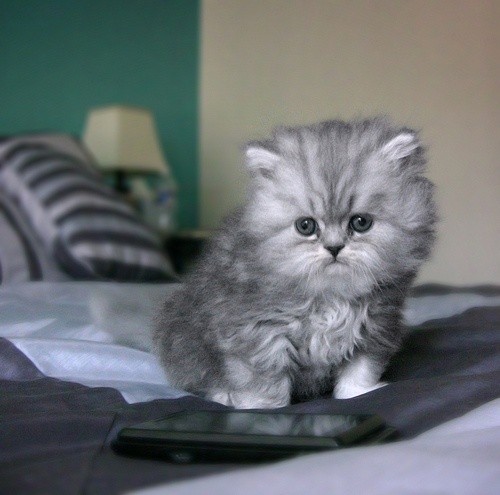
[{"x1": 110, "y1": 408, "x2": 400, "y2": 462}]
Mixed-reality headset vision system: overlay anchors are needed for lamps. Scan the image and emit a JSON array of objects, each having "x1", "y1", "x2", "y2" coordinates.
[{"x1": 82, "y1": 106, "x2": 169, "y2": 212}]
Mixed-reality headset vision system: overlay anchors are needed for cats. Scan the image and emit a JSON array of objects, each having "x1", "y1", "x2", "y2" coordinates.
[{"x1": 150, "y1": 112, "x2": 442, "y2": 411}]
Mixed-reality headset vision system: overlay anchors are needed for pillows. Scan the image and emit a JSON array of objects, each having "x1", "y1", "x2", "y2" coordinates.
[{"x1": 0, "y1": 132, "x2": 180, "y2": 283}]
